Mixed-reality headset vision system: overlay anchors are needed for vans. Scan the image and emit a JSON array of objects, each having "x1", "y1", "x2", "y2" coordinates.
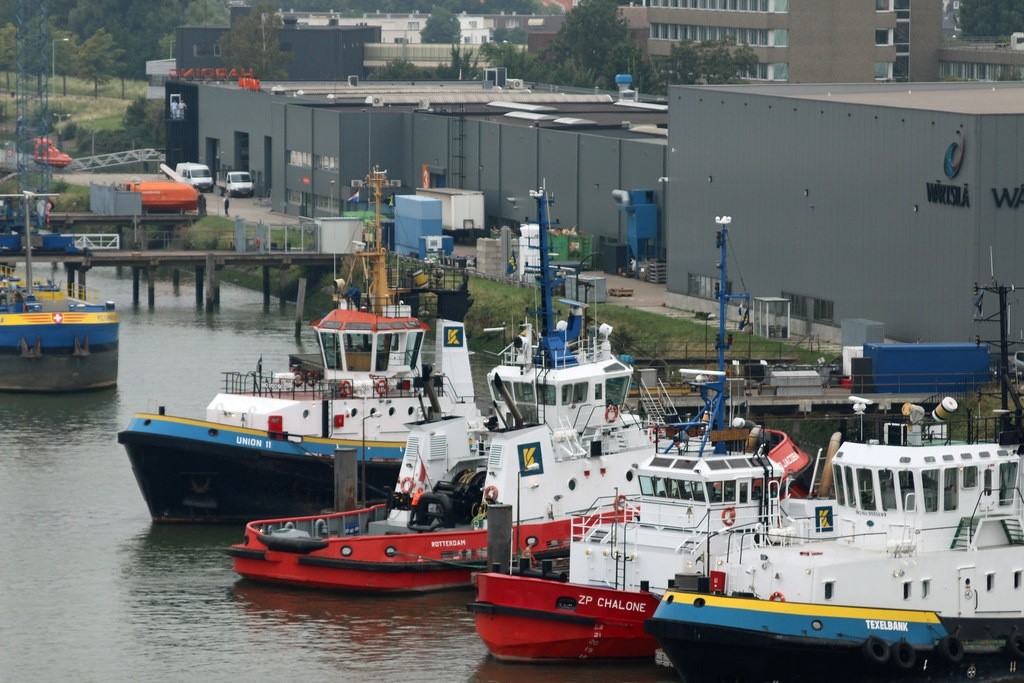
[{"x1": 175, "y1": 162, "x2": 215, "y2": 192}]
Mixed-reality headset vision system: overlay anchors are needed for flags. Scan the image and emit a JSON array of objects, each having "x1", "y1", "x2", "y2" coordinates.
[
  {"x1": 739, "y1": 308, "x2": 749, "y2": 329},
  {"x1": 346, "y1": 188, "x2": 359, "y2": 205},
  {"x1": 508, "y1": 254, "x2": 517, "y2": 273},
  {"x1": 386, "y1": 192, "x2": 396, "y2": 206}
]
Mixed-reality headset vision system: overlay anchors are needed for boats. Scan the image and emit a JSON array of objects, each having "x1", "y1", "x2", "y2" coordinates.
[
  {"x1": 114, "y1": 160, "x2": 1024, "y2": 683},
  {"x1": 0, "y1": 191, "x2": 121, "y2": 393},
  {"x1": 32, "y1": 138, "x2": 72, "y2": 168},
  {"x1": 122, "y1": 178, "x2": 199, "y2": 212}
]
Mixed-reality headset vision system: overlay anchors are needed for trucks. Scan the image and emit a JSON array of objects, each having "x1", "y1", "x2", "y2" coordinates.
[{"x1": 216, "y1": 170, "x2": 255, "y2": 198}]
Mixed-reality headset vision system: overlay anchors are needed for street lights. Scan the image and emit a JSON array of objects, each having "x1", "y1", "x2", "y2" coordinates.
[
  {"x1": 53, "y1": 113, "x2": 72, "y2": 153},
  {"x1": 51, "y1": 37, "x2": 69, "y2": 100}
]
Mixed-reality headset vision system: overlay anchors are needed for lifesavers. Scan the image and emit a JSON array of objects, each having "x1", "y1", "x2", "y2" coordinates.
[
  {"x1": 306, "y1": 372, "x2": 318, "y2": 386},
  {"x1": 939, "y1": 635, "x2": 965, "y2": 664},
  {"x1": 484, "y1": 486, "x2": 498, "y2": 501},
  {"x1": 864, "y1": 635, "x2": 892, "y2": 664},
  {"x1": 893, "y1": 641, "x2": 917, "y2": 669},
  {"x1": 1006, "y1": 632, "x2": 1024, "y2": 657},
  {"x1": 401, "y1": 477, "x2": 414, "y2": 494},
  {"x1": 293, "y1": 372, "x2": 304, "y2": 386},
  {"x1": 613, "y1": 495, "x2": 629, "y2": 515},
  {"x1": 375, "y1": 379, "x2": 388, "y2": 394},
  {"x1": 769, "y1": 592, "x2": 785, "y2": 602},
  {"x1": 605, "y1": 406, "x2": 619, "y2": 423},
  {"x1": 340, "y1": 380, "x2": 352, "y2": 396},
  {"x1": 648, "y1": 427, "x2": 662, "y2": 443},
  {"x1": 721, "y1": 506, "x2": 735, "y2": 526}
]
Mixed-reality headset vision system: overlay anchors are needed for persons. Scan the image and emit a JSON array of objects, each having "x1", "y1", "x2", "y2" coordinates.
[
  {"x1": 224, "y1": 196, "x2": 229, "y2": 215},
  {"x1": 198, "y1": 194, "x2": 207, "y2": 216}
]
